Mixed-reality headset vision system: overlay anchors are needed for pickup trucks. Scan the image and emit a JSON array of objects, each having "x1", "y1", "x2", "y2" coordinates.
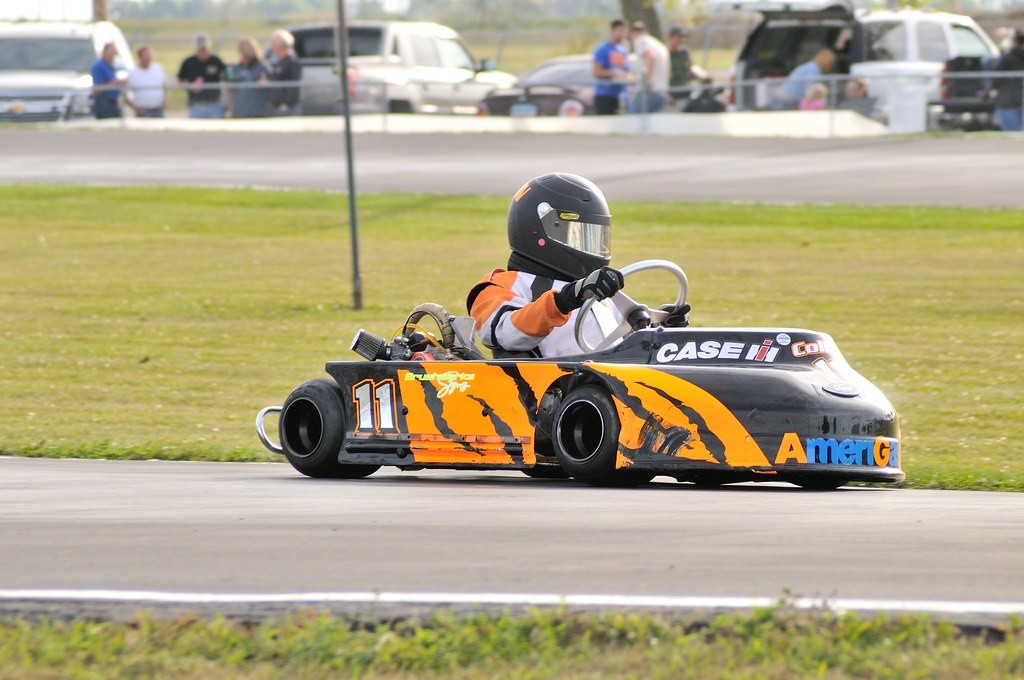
[
  {"x1": 264, "y1": 21, "x2": 518, "y2": 116},
  {"x1": 939, "y1": 56, "x2": 1002, "y2": 130}
]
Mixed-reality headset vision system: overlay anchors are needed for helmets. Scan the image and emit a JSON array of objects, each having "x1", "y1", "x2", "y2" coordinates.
[{"x1": 508, "y1": 173, "x2": 613, "y2": 278}]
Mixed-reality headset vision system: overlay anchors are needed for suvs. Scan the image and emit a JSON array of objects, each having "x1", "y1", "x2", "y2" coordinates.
[{"x1": 0, "y1": 19, "x2": 138, "y2": 121}]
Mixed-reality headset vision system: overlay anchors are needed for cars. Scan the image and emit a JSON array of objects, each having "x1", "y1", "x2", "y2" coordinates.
[{"x1": 476, "y1": 53, "x2": 597, "y2": 116}]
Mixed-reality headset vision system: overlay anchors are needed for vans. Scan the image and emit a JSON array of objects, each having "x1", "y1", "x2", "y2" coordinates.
[{"x1": 715, "y1": 4, "x2": 1000, "y2": 135}]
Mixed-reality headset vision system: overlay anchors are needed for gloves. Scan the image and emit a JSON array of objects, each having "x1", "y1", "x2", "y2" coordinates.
[
  {"x1": 659, "y1": 301, "x2": 690, "y2": 326},
  {"x1": 560, "y1": 266, "x2": 625, "y2": 308}
]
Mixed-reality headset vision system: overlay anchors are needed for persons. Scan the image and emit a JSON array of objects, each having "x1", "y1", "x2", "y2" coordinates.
[
  {"x1": 770, "y1": 49, "x2": 876, "y2": 118},
  {"x1": 592, "y1": 19, "x2": 711, "y2": 114},
  {"x1": 89, "y1": 28, "x2": 302, "y2": 119},
  {"x1": 980, "y1": 25, "x2": 1024, "y2": 131},
  {"x1": 466, "y1": 171, "x2": 691, "y2": 357}
]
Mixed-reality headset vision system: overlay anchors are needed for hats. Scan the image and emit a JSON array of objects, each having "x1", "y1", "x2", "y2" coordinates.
[
  {"x1": 194, "y1": 34, "x2": 212, "y2": 48},
  {"x1": 670, "y1": 28, "x2": 688, "y2": 37}
]
